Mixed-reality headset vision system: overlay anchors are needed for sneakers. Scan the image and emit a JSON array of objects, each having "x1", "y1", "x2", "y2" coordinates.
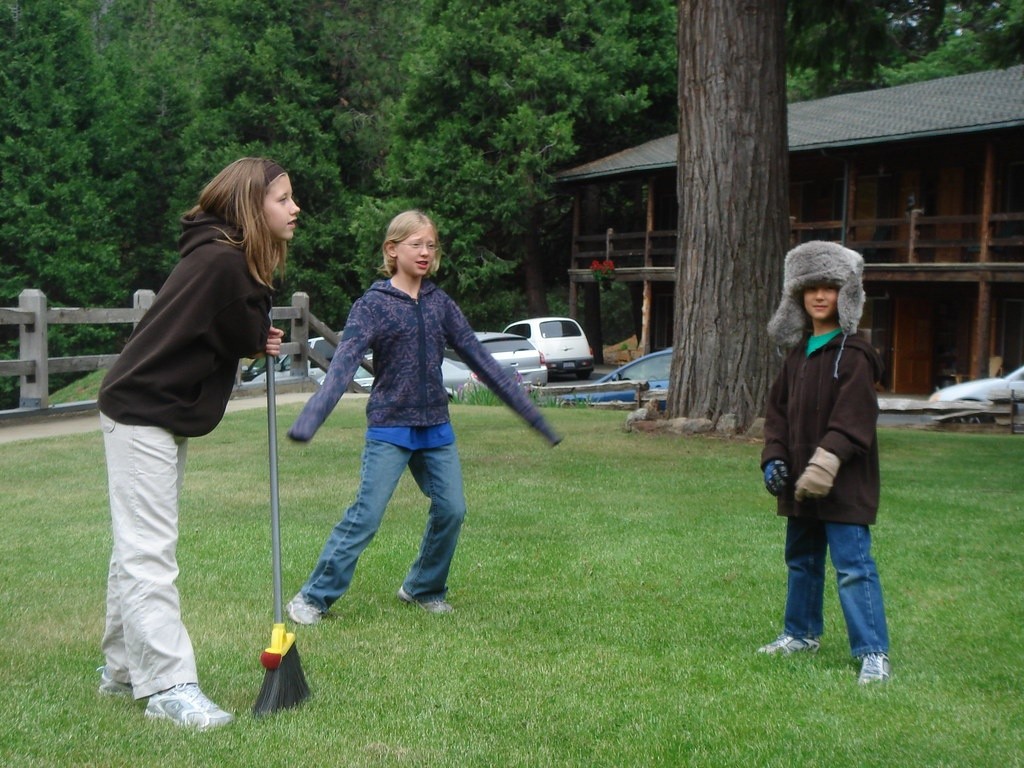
[
  {"x1": 399, "y1": 585, "x2": 457, "y2": 615},
  {"x1": 96, "y1": 664, "x2": 133, "y2": 696},
  {"x1": 757, "y1": 634, "x2": 820, "y2": 654},
  {"x1": 145, "y1": 683, "x2": 233, "y2": 732},
  {"x1": 858, "y1": 652, "x2": 890, "y2": 686},
  {"x1": 285, "y1": 591, "x2": 323, "y2": 625}
]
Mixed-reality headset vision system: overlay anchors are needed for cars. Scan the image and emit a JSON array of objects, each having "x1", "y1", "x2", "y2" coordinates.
[
  {"x1": 928, "y1": 365, "x2": 1024, "y2": 424},
  {"x1": 503, "y1": 318, "x2": 595, "y2": 380},
  {"x1": 241, "y1": 332, "x2": 548, "y2": 402},
  {"x1": 534, "y1": 347, "x2": 672, "y2": 411}
]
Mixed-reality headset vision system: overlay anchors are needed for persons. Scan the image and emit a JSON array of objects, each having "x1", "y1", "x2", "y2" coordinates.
[
  {"x1": 754, "y1": 241, "x2": 892, "y2": 686},
  {"x1": 288, "y1": 209, "x2": 565, "y2": 627},
  {"x1": 96, "y1": 158, "x2": 300, "y2": 732}
]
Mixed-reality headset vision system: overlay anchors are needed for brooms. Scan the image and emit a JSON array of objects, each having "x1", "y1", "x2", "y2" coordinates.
[{"x1": 253, "y1": 296, "x2": 310, "y2": 714}]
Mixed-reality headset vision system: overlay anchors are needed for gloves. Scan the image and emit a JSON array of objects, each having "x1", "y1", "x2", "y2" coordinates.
[
  {"x1": 794, "y1": 446, "x2": 841, "y2": 503},
  {"x1": 764, "y1": 459, "x2": 789, "y2": 498}
]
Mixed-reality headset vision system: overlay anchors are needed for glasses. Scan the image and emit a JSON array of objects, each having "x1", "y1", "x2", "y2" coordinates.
[{"x1": 396, "y1": 240, "x2": 440, "y2": 252}]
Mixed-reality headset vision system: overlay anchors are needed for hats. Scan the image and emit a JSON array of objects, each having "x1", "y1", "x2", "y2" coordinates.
[{"x1": 766, "y1": 239, "x2": 867, "y2": 378}]
